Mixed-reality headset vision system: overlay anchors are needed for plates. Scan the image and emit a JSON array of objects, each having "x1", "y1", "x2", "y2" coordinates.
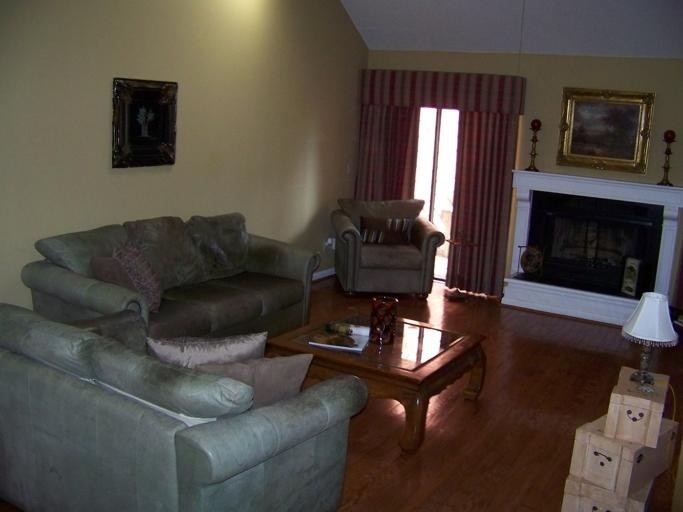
[{"x1": 308, "y1": 324, "x2": 371, "y2": 352}]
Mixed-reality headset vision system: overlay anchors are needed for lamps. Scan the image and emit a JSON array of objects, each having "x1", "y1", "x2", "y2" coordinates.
[
  {"x1": 524, "y1": 119, "x2": 542, "y2": 172},
  {"x1": 621, "y1": 291, "x2": 680, "y2": 386},
  {"x1": 657, "y1": 130, "x2": 676, "y2": 186}
]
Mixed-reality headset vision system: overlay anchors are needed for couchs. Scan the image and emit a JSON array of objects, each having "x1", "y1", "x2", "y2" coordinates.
[
  {"x1": 22, "y1": 212, "x2": 321, "y2": 337},
  {"x1": 0, "y1": 302, "x2": 369, "y2": 510}
]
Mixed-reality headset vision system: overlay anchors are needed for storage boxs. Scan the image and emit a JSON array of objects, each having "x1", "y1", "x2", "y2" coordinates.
[{"x1": 561, "y1": 366, "x2": 678, "y2": 511}]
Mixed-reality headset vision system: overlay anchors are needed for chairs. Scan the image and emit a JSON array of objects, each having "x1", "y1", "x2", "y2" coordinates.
[{"x1": 331, "y1": 199, "x2": 446, "y2": 298}]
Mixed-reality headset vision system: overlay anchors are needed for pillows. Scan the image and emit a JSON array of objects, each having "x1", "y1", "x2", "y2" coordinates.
[
  {"x1": 359, "y1": 215, "x2": 414, "y2": 244},
  {"x1": 89, "y1": 238, "x2": 164, "y2": 315},
  {"x1": 145, "y1": 331, "x2": 313, "y2": 409}
]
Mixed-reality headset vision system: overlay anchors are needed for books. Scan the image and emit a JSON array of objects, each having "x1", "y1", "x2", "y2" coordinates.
[{"x1": 307, "y1": 319, "x2": 372, "y2": 353}]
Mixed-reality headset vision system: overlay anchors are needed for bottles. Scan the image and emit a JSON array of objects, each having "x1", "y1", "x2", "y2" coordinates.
[{"x1": 369, "y1": 296, "x2": 398, "y2": 345}]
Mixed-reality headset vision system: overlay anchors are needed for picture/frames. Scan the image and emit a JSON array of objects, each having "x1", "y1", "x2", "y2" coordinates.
[
  {"x1": 111, "y1": 77, "x2": 178, "y2": 168},
  {"x1": 555, "y1": 86, "x2": 657, "y2": 175}
]
